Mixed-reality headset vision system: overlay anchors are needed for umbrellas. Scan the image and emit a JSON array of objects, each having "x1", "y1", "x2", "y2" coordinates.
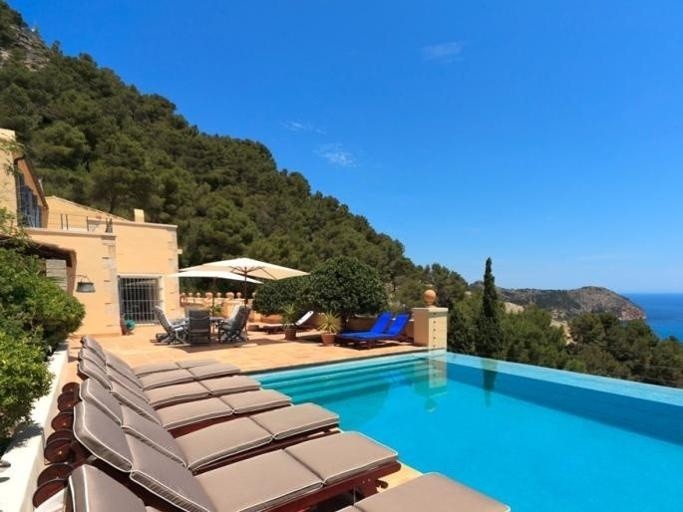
[{"x1": 165, "y1": 258, "x2": 311, "y2": 343}]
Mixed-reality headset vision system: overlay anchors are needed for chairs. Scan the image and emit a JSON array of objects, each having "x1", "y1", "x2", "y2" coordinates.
[
  {"x1": 154, "y1": 305, "x2": 251, "y2": 345},
  {"x1": 340, "y1": 312, "x2": 410, "y2": 349},
  {"x1": 31, "y1": 379, "x2": 511, "y2": 512},
  {"x1": 50, "y1": 336, "x2": 293, "y2": 432},
  {"x1": 259, "y1": 310, "x2": 314, "y2": 333}
]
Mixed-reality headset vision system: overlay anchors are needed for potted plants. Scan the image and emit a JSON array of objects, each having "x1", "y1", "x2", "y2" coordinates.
[
  {"x1": 279, "y1": 303, "x2": 302, "y2": 339},
  {"x1": 315, "y1": 309, "x2": 344, "y2": 346}
]
